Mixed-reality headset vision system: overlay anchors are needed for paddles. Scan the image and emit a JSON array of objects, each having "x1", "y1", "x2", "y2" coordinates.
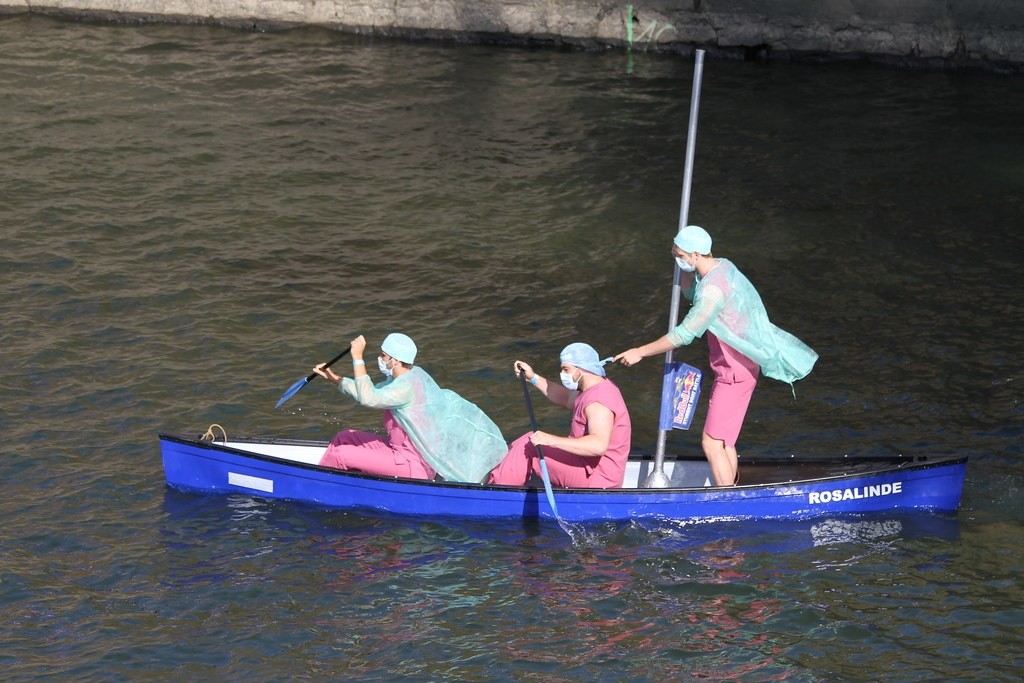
[
  {"x1": 515, "y1": 364, "x2": 562, "y2": 522},
  {"x1": 273, "y1": 344, "x2": 353, "y2": 409}
]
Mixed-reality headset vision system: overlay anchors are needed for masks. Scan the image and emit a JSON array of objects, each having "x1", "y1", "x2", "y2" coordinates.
[
  {"x1": 378, "y1": 357, "x2": 395, "y2": 376},
  {"x1": 675, "y1": 256, "x2": 696, "y2": 272},
  {"x1": 560, "y1": 368, "x2": 582, "y2": 390}
]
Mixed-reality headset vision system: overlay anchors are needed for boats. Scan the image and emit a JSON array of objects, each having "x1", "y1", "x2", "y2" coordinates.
[{"x1": 161, "y1": 429, "x2": 972, "y2": 523}]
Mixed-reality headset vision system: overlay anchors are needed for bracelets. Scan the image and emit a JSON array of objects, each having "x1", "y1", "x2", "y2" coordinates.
[
  {"x1": 529, "y1": 374, "x2": 538, "y2": 385},
  {"x1": 352, "y1": 360, "x2": 364, "y2": 366}
]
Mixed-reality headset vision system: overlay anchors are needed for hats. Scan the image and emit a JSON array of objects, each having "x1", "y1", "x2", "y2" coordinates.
[
  {"x1": 381, "y1": 333, "x2": 417, "y2": 364},
  {"x1": 674, "y1": 225, "x2": 712, "y2": 255},
  {"x1": 560, "y1": 342, "x2": 606, "y2": 375}
]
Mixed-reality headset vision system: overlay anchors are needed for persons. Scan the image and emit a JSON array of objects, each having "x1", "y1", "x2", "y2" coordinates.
[
  {"x1": 612, "y1": 225, "x2": 818, "y2": 487},
  {"x1": 486, "y1": 342, "x2": 631, "y2": 488},
  {"x1": 312, "y1": 333, "x2": 509, "y2": 484}
]
